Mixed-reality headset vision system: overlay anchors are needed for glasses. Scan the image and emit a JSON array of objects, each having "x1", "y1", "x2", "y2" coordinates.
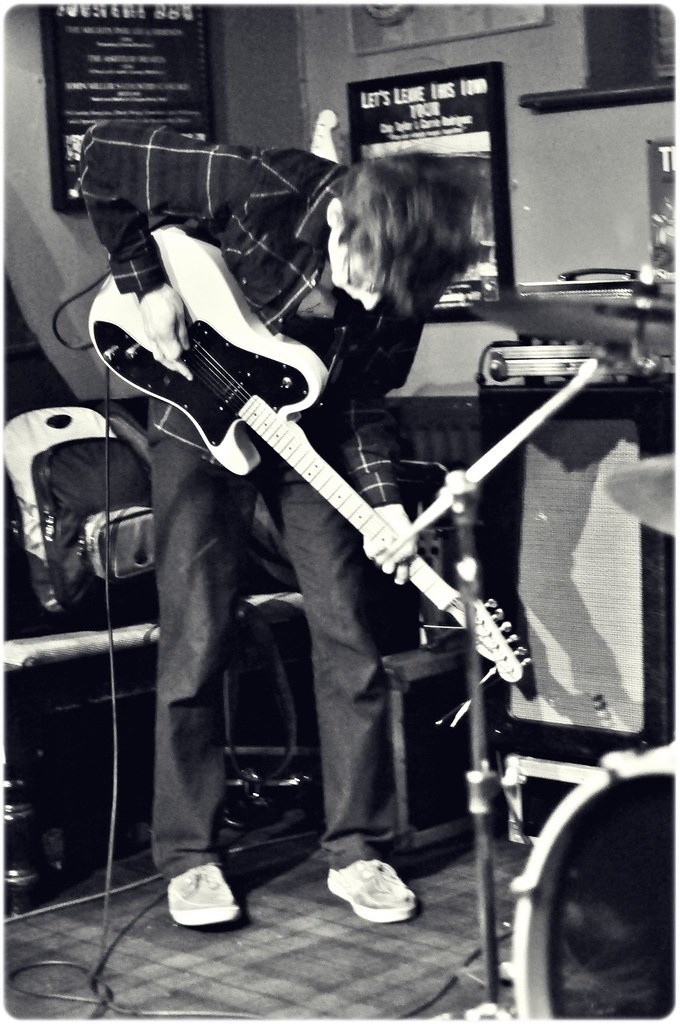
[{"x1": 344, "y1": 241, "x2": 388, "y2": 293}]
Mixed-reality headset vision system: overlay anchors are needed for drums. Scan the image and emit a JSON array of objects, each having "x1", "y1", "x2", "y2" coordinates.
[{"x1": 506, "y1": 737, "x2": 674, "y2": 1020}]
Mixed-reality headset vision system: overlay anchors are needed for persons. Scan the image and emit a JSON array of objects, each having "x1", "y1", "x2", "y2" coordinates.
[{"x1": 78, "y1": 120, "x2": 467, "y2": 924}]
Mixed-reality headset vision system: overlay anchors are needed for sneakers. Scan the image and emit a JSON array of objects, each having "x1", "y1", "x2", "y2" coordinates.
[
  {"x1": 166, "y1": 860, "x2": 238, "y2": 926},
  {"x1": 327, "y1": 859, "x2": 415, "y2": 923}
]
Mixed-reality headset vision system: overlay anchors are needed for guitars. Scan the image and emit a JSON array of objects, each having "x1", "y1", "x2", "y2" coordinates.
[{"x1": 84, "y1": 222, "x2": 537, "y2": 685}]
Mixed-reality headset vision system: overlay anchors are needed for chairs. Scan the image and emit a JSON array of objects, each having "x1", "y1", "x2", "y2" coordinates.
[{"x1": 4, "y1": 393, "x2": 486, "y2": 915}]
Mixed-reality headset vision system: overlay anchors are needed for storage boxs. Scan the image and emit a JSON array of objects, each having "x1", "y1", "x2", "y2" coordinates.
[{"x1": 503, "y1": 755, "x2": 595, "y2": 846}]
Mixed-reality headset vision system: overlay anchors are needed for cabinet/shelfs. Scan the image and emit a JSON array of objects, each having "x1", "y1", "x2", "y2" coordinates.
[{"x1": 475, "y1": 383, "x2": 675, "y2": 769}]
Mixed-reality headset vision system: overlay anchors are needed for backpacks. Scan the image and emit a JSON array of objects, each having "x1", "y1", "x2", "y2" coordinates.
[{"x1": 5, "y1": 402, "x2": 159, "y2": 630}]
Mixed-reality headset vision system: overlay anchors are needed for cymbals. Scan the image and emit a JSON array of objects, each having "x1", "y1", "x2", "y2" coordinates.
[
  {"x1": 456, "y1": 291, "x2": 676, "y2": 356},
  {"x1": 603, "y1": 452, "x2": 675, "y2": 539}
]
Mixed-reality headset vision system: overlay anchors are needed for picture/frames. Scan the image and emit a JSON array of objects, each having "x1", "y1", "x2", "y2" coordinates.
[
  {"x1": 36, "y1": 3, "x2": 216, "y2": 213},
  {"x1": 345, "y1": 59, "x2": 518, "y2": 324}
]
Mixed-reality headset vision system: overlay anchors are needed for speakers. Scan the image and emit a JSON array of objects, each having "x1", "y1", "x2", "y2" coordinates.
[{"x1": 480, "y1": 382, "x2": 676, "y2": 768}]
